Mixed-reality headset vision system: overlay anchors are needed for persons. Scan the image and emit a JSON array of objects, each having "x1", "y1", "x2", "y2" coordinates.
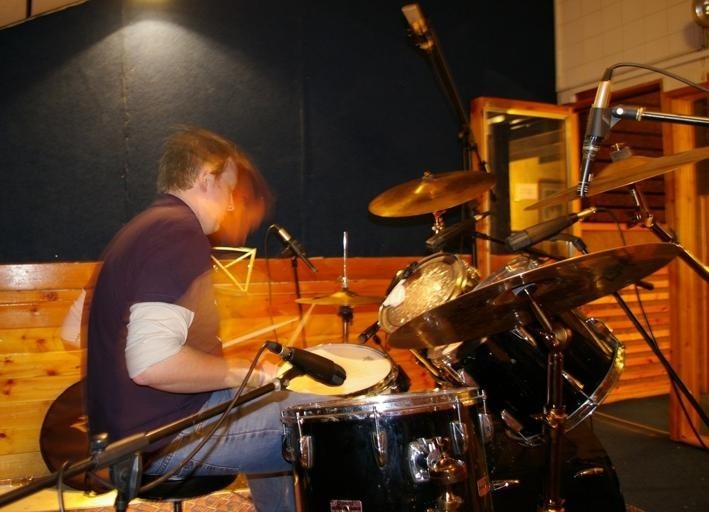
[{"x1": 81, "y1": 125, "x2": 344, "y2": 511}]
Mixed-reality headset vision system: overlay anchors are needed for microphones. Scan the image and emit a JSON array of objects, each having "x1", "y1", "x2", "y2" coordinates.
[
  {"x1": 504, "y1": 207, "x2": 607, "y2": 255},
  {"x1": 573, "y1": 78, "x2": 613, "y2": 198},
  {"x1": 608, "y1": 142, "x2": 661, "y2": 227},
  {"x1": 422, "y1": 213, "x2": 480, "y2": 252},
  {"x1": 257, "y1": 340, "x2": 350, "y2": 390},
  {"x1": 273, "y1": 224, "x2": 324, "y2": 275},
  {"x1": 355, "y1": 315, "x2": 382, "y2": 345},
  {"x1": 401, "y1": 3, "x2": 436, "y2": 54}
]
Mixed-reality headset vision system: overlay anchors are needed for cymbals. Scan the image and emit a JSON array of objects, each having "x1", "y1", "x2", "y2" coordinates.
[
  {"x1": 524, "y1": 142, "x2": 709, "y2": 212},
  {"x1": 293, "y1": 288, "x2": 386, "y2": 306},
  {"x1": 368, "y1": 170, "x2": 498, "y2": 217},
  {"x1": 389, "y1": 241, "x2": 684, "y2": 349}
]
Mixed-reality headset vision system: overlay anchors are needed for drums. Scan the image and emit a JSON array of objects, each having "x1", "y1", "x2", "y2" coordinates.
[
  {"x1": 487, "y1": 421, "x2": 627, "y2": 512},
  {"x1": 281, "y1": 386, "x2": 494, "y2": 512},
  {"x1": 277, "y1": 342, "x2": 400, "y2": 399},
  {"x1": 428, "y1": 255, "x2": 626, "y2": 448},
  {"x1": 378, "y1": 252, "x2": 481, "y2": 335}
]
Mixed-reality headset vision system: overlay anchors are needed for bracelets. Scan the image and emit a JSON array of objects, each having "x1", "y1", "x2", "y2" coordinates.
[{"x1": 258, "y1": 370, "x2": 265, "y2": 386}]
